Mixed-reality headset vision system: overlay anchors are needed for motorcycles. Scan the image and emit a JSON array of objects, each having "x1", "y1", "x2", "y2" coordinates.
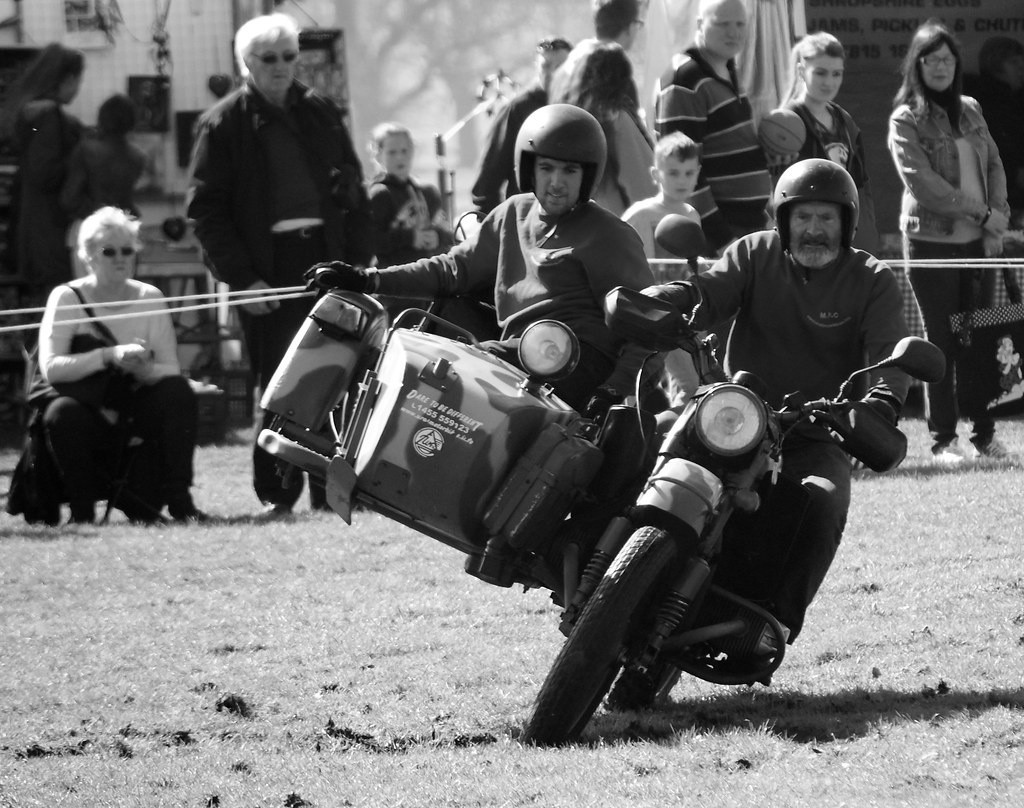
[{"x1": 252, "y1": 210, "x2": 945, "y2": 747}]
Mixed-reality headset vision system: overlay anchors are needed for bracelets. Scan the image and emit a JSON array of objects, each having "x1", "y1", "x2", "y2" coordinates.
[{"x1": 979, "y1": 206, "x2": 992, "y2": 226}]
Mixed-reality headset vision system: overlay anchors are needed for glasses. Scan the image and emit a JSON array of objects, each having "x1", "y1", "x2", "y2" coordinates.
[
  {"x1": 250, "y1": 48, "x2": 300, "y2": 64},
  {"x1": 633, "y1": 19, "x2": 644, "y2": 29},
  {"x1": 920, "y1": 55, "x2": 956, "y2": 67},
  {"x1": 101, "y1": 247, "x2": 134, "y2": 256}
]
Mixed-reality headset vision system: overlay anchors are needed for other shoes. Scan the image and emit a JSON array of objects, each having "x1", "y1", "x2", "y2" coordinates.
[
  {"x1": 975, "y1": 440, "x2": 1018, "y2": 466},
  {"x1": 754, "y1": 621, "x2": 791, "y2": 659},
  {"x1": 168, "y1": 496, "x2": 209, "y2": 524},
  {"x1": 62, "y1": 502, "x2": 96, "y2": 531},
  {"x1": 261, "y1": 504, "x2": 294, "y2": 521},
  {"x1": 933, "y1": 443, "x2": 966, "y2": 465}
]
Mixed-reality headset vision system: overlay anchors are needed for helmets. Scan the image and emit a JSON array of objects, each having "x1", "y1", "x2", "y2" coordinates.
[
  {"x1": 773, "y1": 158, "x2": 860, "y2": 256},
  {"x1": 513, "y1": 104, "x2": 607, "y2": 204}
]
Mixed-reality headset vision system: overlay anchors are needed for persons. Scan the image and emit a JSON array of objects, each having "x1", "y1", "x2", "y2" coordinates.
[
  {"x1": 302, "y1": 103, "x2": 671, "y2": 440},
  {"x1": 26, "y1": 203, "x2": 208, "y2": 524},
  {"x1": 366, "y1": 120, "x2": 463, "y2": 269},
  {"x1": 471, "y1": 36, "x2": 572, "y2": 224},
  {"x1": 620, "y1": 0, "x2": 800, "y2": 409},
  {"x1": 639, "y1": 159, "x2": 912, "y2": 646},
  {"x1": 548, "y1": 0, "x2": 660, "y2": 219},
  {"x1": 964, "y1": 36, "x2": 1024, "y2": 232},
  {"x1": 887, "y1": 19, "x2": 1011, "y2": 460},
  {"x1": 771, "y1": 31, "x2": 881, "y2": 260},
  {"x1": 0, "y1": 44, "x2": 145, "y2": 344},
  {"x1": 186, "y1": 13, "x2": 375, "y2": 512}
]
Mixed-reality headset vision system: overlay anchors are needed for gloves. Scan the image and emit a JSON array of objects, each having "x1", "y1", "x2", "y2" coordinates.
[
  {"x1": 861, "y1": 398, "x2": 898, "y2": 426},
  {"x1": 581, "y1": 394, "x2": 613, "y2": 426},
  {"x1": 302, "y1": 261, "x2": 378, "y2": 302},
  {"x1": 640, "y1": 284, "x2": 690, "y2": 311}
]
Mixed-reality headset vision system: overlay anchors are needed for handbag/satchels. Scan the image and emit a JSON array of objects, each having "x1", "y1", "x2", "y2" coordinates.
[{"x1": 26, "y1": 284, "x2": 131, "y2": 407}]
